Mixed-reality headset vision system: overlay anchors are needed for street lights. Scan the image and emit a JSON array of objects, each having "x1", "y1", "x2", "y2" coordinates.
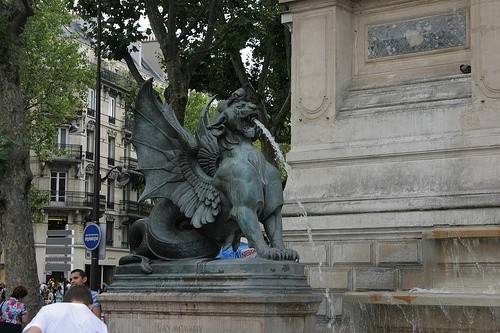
[{"x1": 88, "y1": 3, "x2": 130, "y2": 289}]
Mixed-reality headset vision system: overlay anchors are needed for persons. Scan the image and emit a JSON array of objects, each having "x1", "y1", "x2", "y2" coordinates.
[
  {"x1": 22, "y1": 285, "x2": 109, "y2": 333},
  {"x1": 69, "y1": 269, "x2": 101, "y2": 319},
  {"x1": 0, "y1": 286, "x2": 29, "y2": 333},
  {"x1": 39, "y1": 269, "x2": 72, "y2": 305},
  {"x1": 0, "y1": 283, "x2": 6, "y2": 303}
]
